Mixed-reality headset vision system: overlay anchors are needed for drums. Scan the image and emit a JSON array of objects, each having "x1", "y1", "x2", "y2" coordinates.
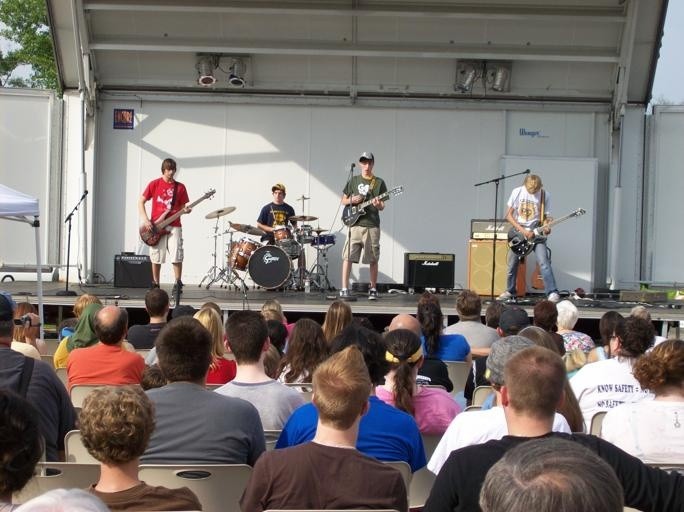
[
  {"x1": 295, "y1": 224, "x2": 318, "y2": 244},
  {"x1": 247, "y1": 245, "x2": 291, "y2": 289},
  {"x1": 273, "y1": 225, "x2": 294, "y2": 242},
  {"x1": 311, "y1": 234, "x2": 335, "y2": 249},
  {"x1": 238, "y1": 238, "x2": 263, "y2": 258},
  {"x1": 228, "y1": 241, "x2": 248, "y2": 270}
]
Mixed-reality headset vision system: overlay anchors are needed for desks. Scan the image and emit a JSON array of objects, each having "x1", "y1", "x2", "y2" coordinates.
[{"x1": 468, "y1": 239, "x2": 526, "y2": 297}]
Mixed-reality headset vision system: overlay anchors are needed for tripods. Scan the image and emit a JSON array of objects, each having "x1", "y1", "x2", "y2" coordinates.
[
  {"x1": 199, "y1": 236, "x2": 223, "y2": 289},
  {"x1": 308, "y1": 247, "x2": 333, "y2": 292},
  {"x1": 282, "y1": 236, "x2": 323, "y2": 292},
  {"x1": 207, "y1": 247, "x2": 249, "y2": 291}
]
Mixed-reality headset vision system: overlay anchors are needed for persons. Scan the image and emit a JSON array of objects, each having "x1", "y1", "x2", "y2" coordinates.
[
  {"x1": 496, "y1": 175, "x2": 560, "y2": 303},
  {"x1": 256, "y1": 183, "x2": 297, "y2": 292},
  {"x1": 340, "y1": 151, "x2": 390, "y2": 300},
  {"x1": 0, "y1": 289, "x2": 683, "y2": 512},
  {"x1": 139, "y1": 158, "x2": 192, "y2": 297}
]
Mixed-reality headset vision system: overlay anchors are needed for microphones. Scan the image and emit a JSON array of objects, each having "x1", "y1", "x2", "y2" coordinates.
[
  {"x1": 350, "y1": 163, "x2": 355, "y2": 170},
  {"x1": 82, "y1": 189, "x2": 89, "y2": 199},
  {"x1": 523, "y1": 168, "x2": 530, "y2": 174}
]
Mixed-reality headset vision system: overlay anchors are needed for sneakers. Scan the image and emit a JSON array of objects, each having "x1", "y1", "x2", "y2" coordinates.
[
  {"x1": 339, "y1": 289, "x2": 349, "y2": 297},
  {"x1": 368, "y1": 289, "x2": 377, "y2": 300},
  {"x1": 496, "y1": 291, "x2": 517, "y2": 301},
  {"x1": 547, "y1": 292, "x2": 560, "y2": 302}
]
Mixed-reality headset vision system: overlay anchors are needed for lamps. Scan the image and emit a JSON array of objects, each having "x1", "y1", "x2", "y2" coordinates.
[
  {"x1": 456, "y1": 69, "x2": 476, "y2": 91},
  {"x1": 492, "y1": 67, "x2": 507, "y2": 92},
  {"x1": 229, "y1": 57, "x2": 246, "y2": 89},
  {"x1": 195, "y1": 56, "x2": 216, "y2": 87}
]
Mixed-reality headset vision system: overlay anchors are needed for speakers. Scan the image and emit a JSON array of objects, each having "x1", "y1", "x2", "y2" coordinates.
[
  {"x1": 404, "y1": 252, "x2": 456, "y2": 288},
  {"x1": 113, "y1": 255, "x2": 153, "y2": 288}
]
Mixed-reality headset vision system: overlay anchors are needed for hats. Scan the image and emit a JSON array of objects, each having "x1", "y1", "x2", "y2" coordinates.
[
  {"x1": 359, "y1": 152, "x2": 374, "y2": 162},
  {"x1": 272, "y1": 183, "x2": 285, "y2": 193}
]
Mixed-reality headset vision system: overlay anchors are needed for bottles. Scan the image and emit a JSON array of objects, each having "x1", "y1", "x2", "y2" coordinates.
[{"x1": 304, "y1": 276, "x2": 311, "y2": 293}]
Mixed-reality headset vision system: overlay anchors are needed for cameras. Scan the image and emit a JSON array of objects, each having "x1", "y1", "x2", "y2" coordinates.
[{"x1": 13, "y1": 316, "x2": 26, "y2": 325}]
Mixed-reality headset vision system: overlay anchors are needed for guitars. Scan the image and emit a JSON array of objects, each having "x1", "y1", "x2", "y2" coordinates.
[
  {"x1": 139, "y1": 188, "x2": 217, "y2": 245},
  {"x1": 507, "y1": 208, "x2": 585, "y2": 258},
  {"x1": 343, "y1": 186, "x2": 402, "y2": 226}
]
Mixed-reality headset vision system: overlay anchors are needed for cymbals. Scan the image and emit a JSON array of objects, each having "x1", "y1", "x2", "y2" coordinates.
[
  {"x1": 206, "y1": 207, "x2": 235, "y2": 219},
  {"x1": 287, "y1": 216, "x2": 318, "y2": 221},
  {"x1": 310, "y1": 229, "x2": 329, "y2": 232},
  {"x1": 230, "y1": 223, "x2": 268, "y2": 236}
]
